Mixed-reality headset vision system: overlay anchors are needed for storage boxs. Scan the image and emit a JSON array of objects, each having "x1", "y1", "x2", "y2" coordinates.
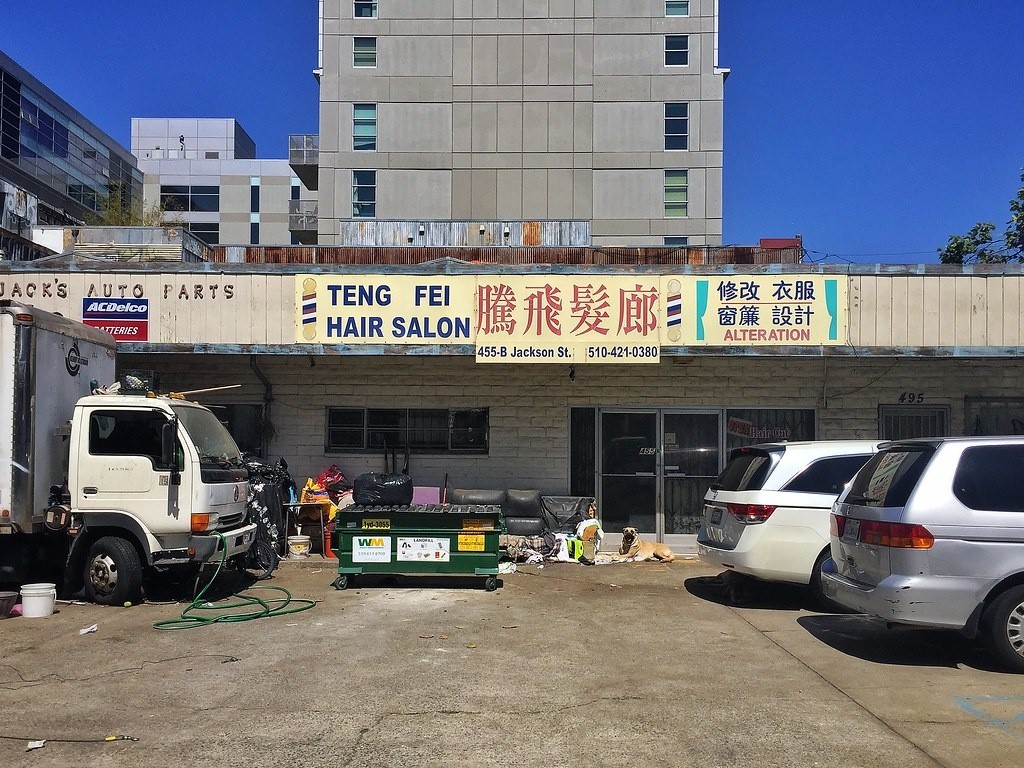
[{"x1": 570, "y1": 541, "x2": 594, "y2": 559}]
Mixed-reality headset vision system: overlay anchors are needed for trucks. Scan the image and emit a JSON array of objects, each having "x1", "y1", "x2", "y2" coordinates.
[{"x1": 0, "y1": 296, "x2": 260, "y2": 606}]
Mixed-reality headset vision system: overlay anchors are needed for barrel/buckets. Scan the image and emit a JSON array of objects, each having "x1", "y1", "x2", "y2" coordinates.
[
  {"x1": 0, "y1": 592, "x2": 18, "y2": 619},
  {"x1": 20, "y1": 583, "x2": 57, "y2": 617},
  {"x1": 287, "y1": 535, "x2": 312, "y2": 559},
  {"x1": 324, "y1": 530, "x2": 338, "y2": 558}
]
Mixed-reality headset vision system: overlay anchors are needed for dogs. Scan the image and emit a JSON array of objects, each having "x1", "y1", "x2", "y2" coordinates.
[{"x1": 606, "y1": 527, "x2": 675, "y2": 563}]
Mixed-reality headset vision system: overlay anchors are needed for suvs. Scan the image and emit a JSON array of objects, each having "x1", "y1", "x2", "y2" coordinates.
[
  {"x1": 818, "y1": 433, "x2": 1024, "y2": 675},
  {"x1": 696, "y1": 436, "x2": 901, "y2": 615}
]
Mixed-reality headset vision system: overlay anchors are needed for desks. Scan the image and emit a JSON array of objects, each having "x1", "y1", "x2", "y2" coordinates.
[{"x1": 283, "y1": 503, "x2": 330, "y2": 560}]
